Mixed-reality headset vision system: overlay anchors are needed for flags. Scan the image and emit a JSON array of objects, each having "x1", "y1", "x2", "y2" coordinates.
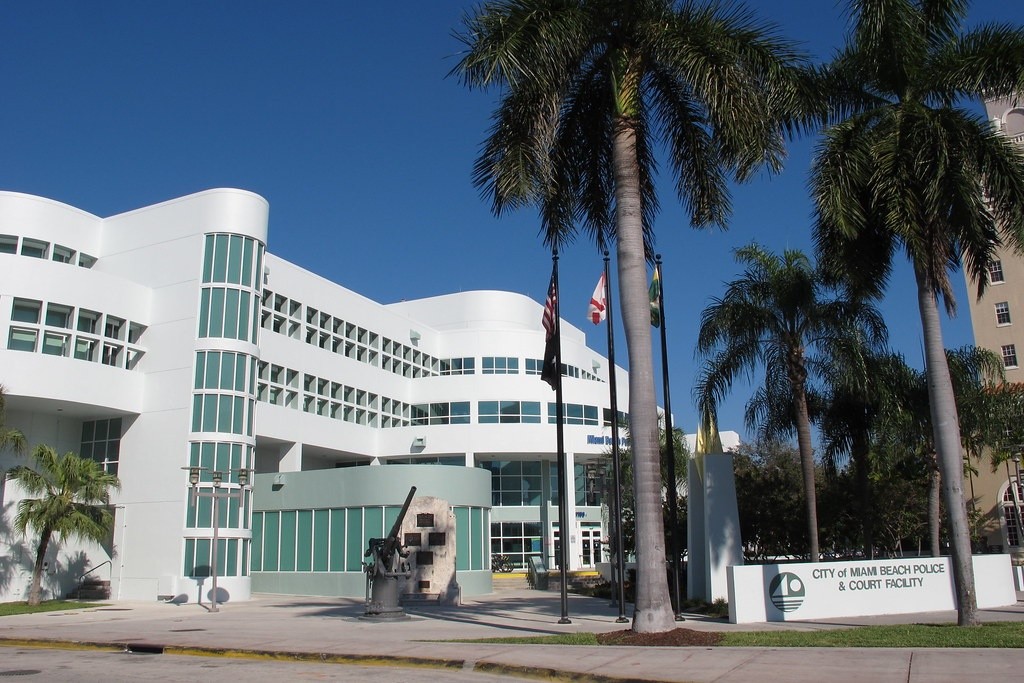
[
  {"x1": 648, "y1": 266, "x2": 661, "y2": 328},
  {"x1": 586, "y1": 271, "x2": 606, "y2": 325},
  {"x1": 541, "y1": 270, "x2": 556, "y2": 391}
]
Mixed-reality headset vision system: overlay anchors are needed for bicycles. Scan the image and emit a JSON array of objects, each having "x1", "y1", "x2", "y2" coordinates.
[{"x1": 491, "y1": 553, "x2": 515, "y2": 572}]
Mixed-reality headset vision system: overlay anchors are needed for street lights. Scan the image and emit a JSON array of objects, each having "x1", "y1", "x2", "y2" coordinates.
[{"x1": 181, "y1": 467, "x2": 257, "y2": 612}]
